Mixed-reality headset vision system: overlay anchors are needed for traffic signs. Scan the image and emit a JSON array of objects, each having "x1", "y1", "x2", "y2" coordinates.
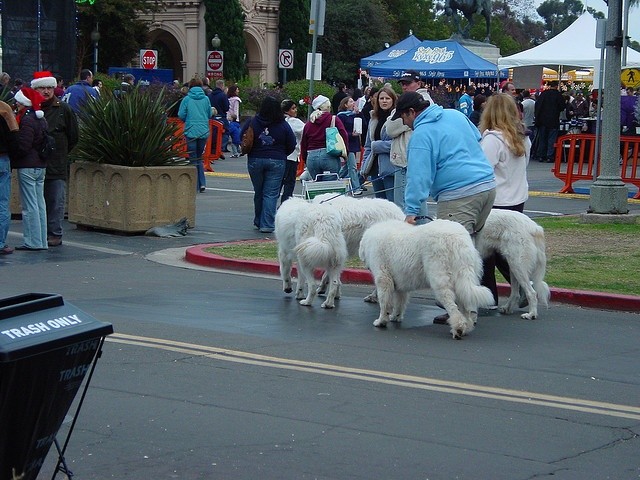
[
  {"x1": 206, "y1": 51, "x2": 224, "y2": 78},
  {"x1": 140, "y1": 49, "x2": 158, "y2": 70}
]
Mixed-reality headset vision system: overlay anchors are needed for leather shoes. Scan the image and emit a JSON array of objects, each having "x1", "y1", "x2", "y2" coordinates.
[
  {"x1": 48, "y1": 236, "x2": 62, "y2": 246},
  {"x1": 433, "y1": 314, "x2": 450, "y2": 325},
  {"x1": 15, "y1": 245, "x2": 41, "y2": 251},
  {"x1": 0, "y1": 245, "x2": 14, "y2": 255}
]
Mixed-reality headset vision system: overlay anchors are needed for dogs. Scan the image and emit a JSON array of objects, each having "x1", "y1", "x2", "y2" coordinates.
[
  {"x1": 273, "y1": 197, "x2": 347, "y2": 308},
  {"x1": 471, "y1": 208, "x2": 550, "y2": 321},
  {"x1": 312, "y1": 192, "x2": 406, "y2": 303},
  {"x1": 358, "y1": 219, "x2": 495, "y2": 340}
]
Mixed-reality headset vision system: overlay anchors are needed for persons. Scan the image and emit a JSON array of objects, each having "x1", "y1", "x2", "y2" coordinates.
[
  {"x1": 92, "y1": 79, "x2": 103, "y2": 97},
  {"x1": 337, "y1": 96, "x2": 368, "y2": 195},
  {"x1": 447, "y1": 85, "x2": 458, "y2": 102},
  {"x1": 358, "y1": 69, "x2": 435, "y2": 226},
  {"x1": 384, "y1": 82, "x2": 391, "y2": 88},
  {"x1": 225, "y1": 113, "x2": 243, "y2": 158},
  {"x1": 30, "y1": 71, "x2": 79, "y2": 247},
  {"x1": 352, "y1": 89, "x2": 362, "y2": 107},
  {"x1": 358, "y1": 98, "x2": 377, "y2": 191},
  {"x1": 180, "y1": 83, "x2": 190, "y2": 94},
  {"x1": 437, "y1": 79, "x2": 446, "y2": 87},
  {"x1": 275, "y1": 98, "x2": 306, "y2": 210},
  {"x1": 532, "y1": 79, "x2": 566, "y2": 163},
  {"x1": 203, "y1": 76, "x2": 213, "y2": 94},
  {"x1": 502, "y1": 81, "x2": 517, "y2": 96},
  {"x1": 390, "y1": 90, "x2": 497, "y2": 325},
  {"x1": 59, "y1": 68, "x2": 100, "y2": 114},
  {"x1": 361, "y1": 86, "x2": 379, "y2": 148},
  {"x1": 300, "y1": 94, "x2": 350, "y2": 182},
  {"x1": 0, "y1": 99, "x2": 20, "y2": 255},
  {"x1": 619, "y1": 84, "x2": 640, "y2": 166},
  {"x1": 516, "y1": 89, "x2": 525, "y2": 113},
  {"x1": 567, "y1": 77, "x2": 601, "y2": 121},
  {"x1": 114, "y1": 73, "x2": 136, "y2": 100},
  {"x1": 211, "y1": 106, "x2": 226, "y2": 160},
  {"x1": 208, "y1": 79, "x2": 230, "y2": 152},
  {"x1": 478, "y1": 93, "x2": 533, "y2": 311},
  {"x1": 226, "y1": 85, "x2": 242, "y2": 152},
  {"x1": 332, "y1": 83, "x2": 348, "y2": 115},
  {"x1": 359, "y1": 86, "x2": 398, "y2": 203},
  {"x1": 357, "y1": 86, "x2": 372, "y2": 112},
  {"x1": 469, "y1": 94, "x2": 489, "y2": 127},
  {"x1": 239, "y1": 95, "x2": 297, "y2": 233},
  {"x1": 177, "y1": 78, "x2": 217, "y2": 193},
  {"x1": 54, "y1": 75, "x2": 64, "y2": 98},
  {"x1": 459, "y1": 86, "x2": 475, "y2": 118},
  {"x1": 4, "y1": 77, "x2": 24, "y2": 115},
  {"x1": 173, "y1": 79, "x2": 179, "y2": 88},
  {"x1": 9, "y1": 87, "x2": 51, "y2": 251},
  {"x1": 459, "y1": 83, "x2": 468, "y2": 99},
  {"x1": 519, "y1": 90, "x2": 536, "y2": 139}
]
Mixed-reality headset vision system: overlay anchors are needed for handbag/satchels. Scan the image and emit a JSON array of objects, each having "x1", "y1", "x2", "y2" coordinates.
[
  {"x1": 326, "y1": 114, "x2": 349, "y2": 159},
  {"x1": 240, "y1": 118, "x2": 255, "y2": 154}
]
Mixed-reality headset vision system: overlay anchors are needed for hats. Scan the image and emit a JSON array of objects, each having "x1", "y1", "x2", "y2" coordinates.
[
  {"x1": 397, "y1": 70, "x2": 421, "y2": 84},
  {"x1": 30, "y1": 70, "x2": 58, "y2": 90},
  {"x1": 391, "y1": 91, "x2": 424, "y2": 121},
  {"x1": 14, "y1": 87, "x2": 47, "y2": 119},
  {"x1": 311, "y1": 95, "x2": 330, "y2": 110}
]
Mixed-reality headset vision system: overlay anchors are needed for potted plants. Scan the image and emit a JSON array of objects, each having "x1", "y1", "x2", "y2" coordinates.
[{"x1": 68, "y1": 77, "x2": 199, "y2": 231}]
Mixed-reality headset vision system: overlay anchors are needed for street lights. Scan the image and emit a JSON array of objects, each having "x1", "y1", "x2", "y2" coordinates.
[
  {"x1": 91, "y1": 22, "x2": 100, "y2": 74},
  {"x1": 282, "y1": 38, "x2": 293, "y2": 85},
  {"x1": 210, "y1": 34, "x2": 222, "y2": 91}
]
